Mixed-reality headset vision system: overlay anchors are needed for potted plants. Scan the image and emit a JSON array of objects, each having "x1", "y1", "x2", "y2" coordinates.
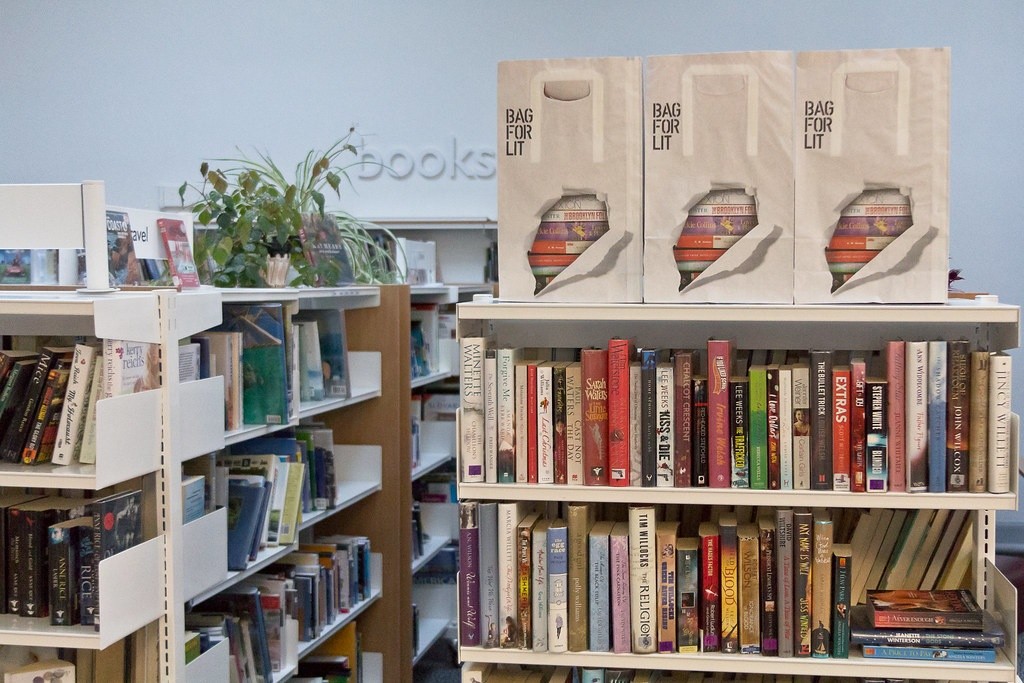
[{"x1": 147, "y1": 125, "x2": 408, "y2": 284}]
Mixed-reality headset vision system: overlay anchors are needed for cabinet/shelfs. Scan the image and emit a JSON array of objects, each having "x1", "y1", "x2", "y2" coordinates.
[
  {"x1": 453, "y1": 294, "x2": 1021, "y2": 682},
  {"x1": 0, "y1": 180, "x2": 499, "y2": 682}
]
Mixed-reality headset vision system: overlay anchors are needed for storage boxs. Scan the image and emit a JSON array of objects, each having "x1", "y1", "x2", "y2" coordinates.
[{"x1": 496, "y1": 46, "x2": 952, "y2": 306}]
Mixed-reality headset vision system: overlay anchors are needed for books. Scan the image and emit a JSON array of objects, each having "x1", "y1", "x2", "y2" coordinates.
[
  {"x1": 0, "y1": 302, "x2": 371, "y2": 682},
  {"x1": 105, "y1": 210, "x2": 498, "y2": 286},
  {"x1": 526, "y1": 189, "x2": 913, "y2": 293},
  {"x1": 410, "y1": 303, "x2": 1011, "y2": 683}
]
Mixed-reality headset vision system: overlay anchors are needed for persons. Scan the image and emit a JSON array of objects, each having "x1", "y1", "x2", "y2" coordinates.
[{"x1": 793, "y1": 409, "x2": 809, "y2": 436}]
